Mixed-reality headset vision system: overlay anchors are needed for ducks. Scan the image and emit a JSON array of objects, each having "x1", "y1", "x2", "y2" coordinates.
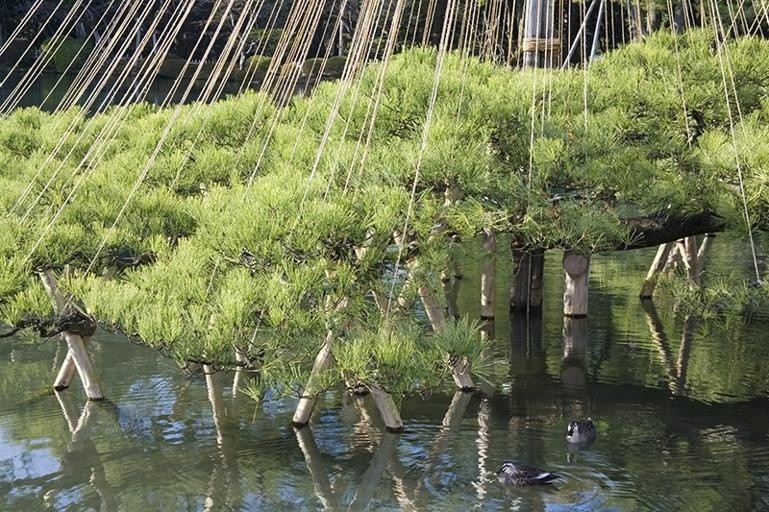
[
  {"x1": 563, "y1": 417, "x2": 596, "y2": 444},
  {"x1": 493, "y1": 461, "x2": 564, "y2": 486}
]
